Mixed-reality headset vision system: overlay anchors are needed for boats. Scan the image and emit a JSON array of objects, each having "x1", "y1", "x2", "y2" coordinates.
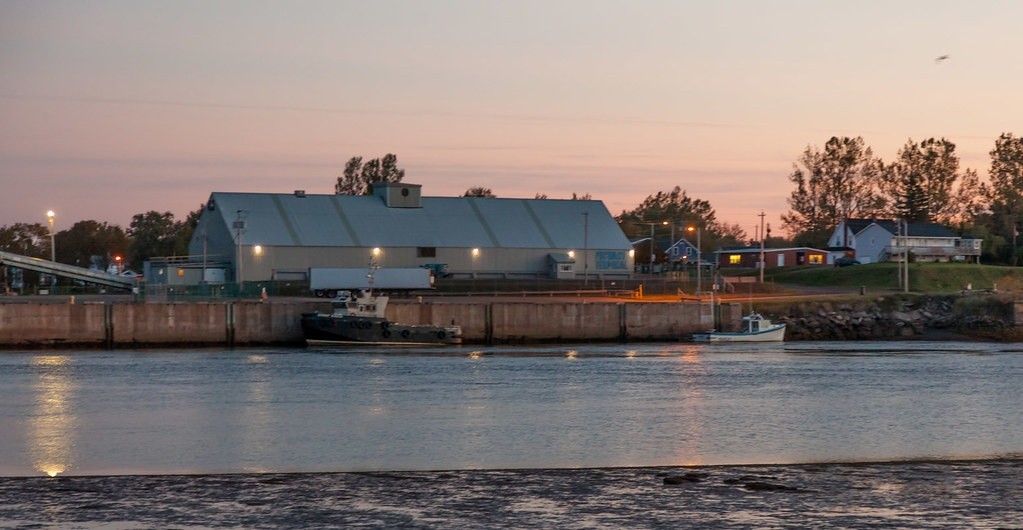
[
  {"x1": 299, "y1": 257, "x2": 464, "y2": 348},
  {"x1": 687, "y1": 287, "x2": 787, "y2": 342}
]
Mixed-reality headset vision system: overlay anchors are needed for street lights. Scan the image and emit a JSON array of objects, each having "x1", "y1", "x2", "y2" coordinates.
[{"x1": 48, "y1": 211, "x2": 56, "y2": 261}]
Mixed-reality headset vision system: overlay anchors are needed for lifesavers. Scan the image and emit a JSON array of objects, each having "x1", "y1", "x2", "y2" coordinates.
[
  {"x1": 381, "y1": 322, "x2": 388, "y2": 329},
  {"x1": 325, "y1": 317, "x2": 336, "y2": 328},
  {"x1": 350, "y1": 312, "x2": 356, "y2": 316},
  {"x1": 383, "y1": 330, "x2": 391, "y2": 338},
  {"x1": 402, "y1": 330, "x2": 409, "y2": 338},
  {"x1": 366, "y1": 321, "x2": 372, "y2": 329},
  {"x1": 350, "y1": 320, "x2": 359, "y2": 328},
  {"x1": 359, "y1": 321, "x2": 366, "y2": 329},
  {"x1": 438, "y1": 331, "x2": 445, "y2": 339}
]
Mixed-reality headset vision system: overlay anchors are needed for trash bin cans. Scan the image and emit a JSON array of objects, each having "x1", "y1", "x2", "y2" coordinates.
[
  {"x1": 448, "y1": 325, "x2": 462, "y2": 344},
  {"x1": 756, "y1": 262, "x2": 766, "y2": 268},
  {"x1": 666, "y1": 271, "x2": 690, "y2": 282}
]
